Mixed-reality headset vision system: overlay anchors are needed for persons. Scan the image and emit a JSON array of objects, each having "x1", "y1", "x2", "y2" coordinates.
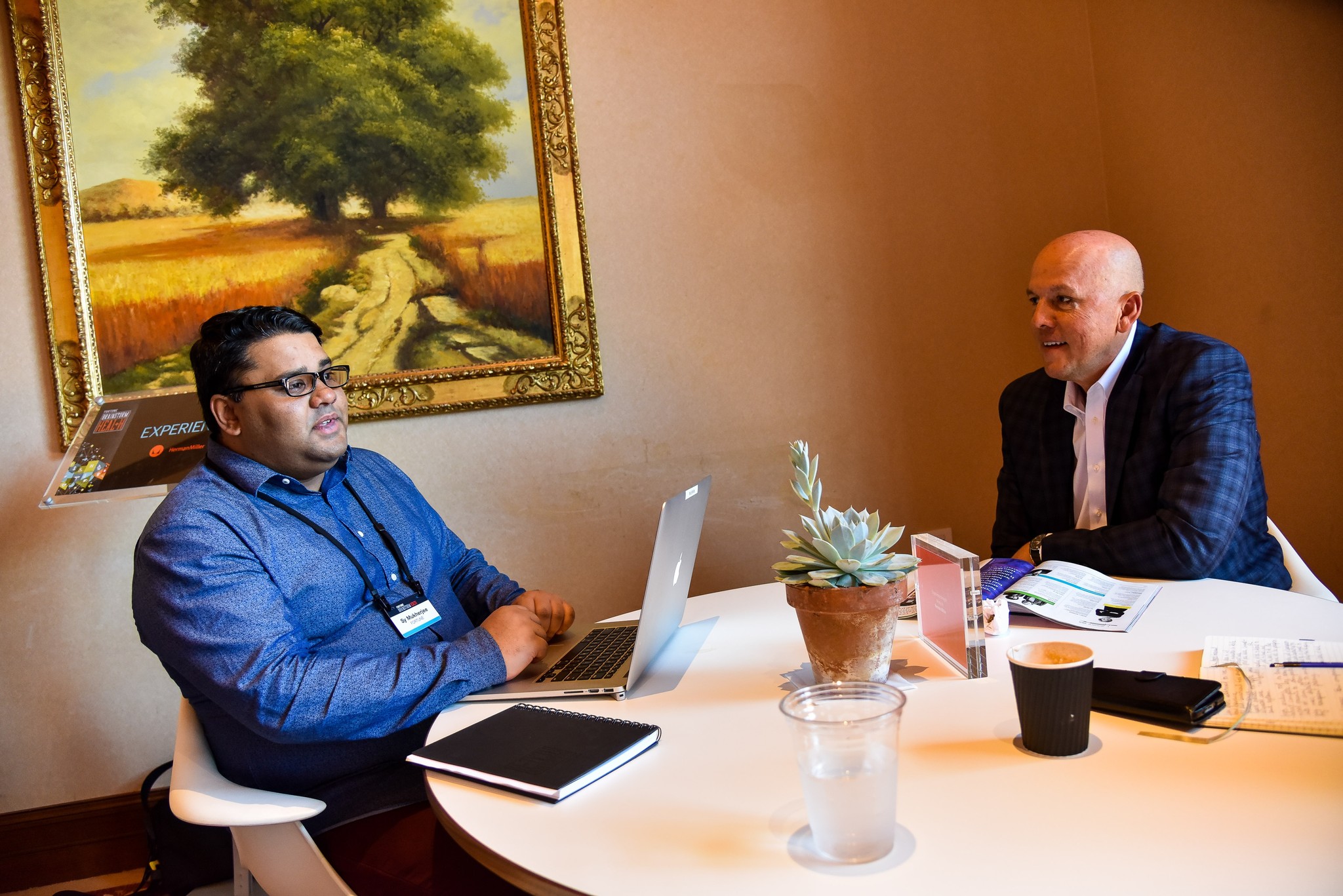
[
  {"x1": 130, "y1": 302, "x2": 577, "y2": 896},
  {"x1": 989, "y1": 228, "x2": 1295, "y2": 594}
]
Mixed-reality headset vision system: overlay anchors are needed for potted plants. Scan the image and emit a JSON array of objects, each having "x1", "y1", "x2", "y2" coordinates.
[{"x1": 769, "y1": 438, "x2": 924, "y2": 689}]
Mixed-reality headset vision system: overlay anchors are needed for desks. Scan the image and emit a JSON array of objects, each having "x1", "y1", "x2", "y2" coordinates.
[{"x1": 423, "y1": 567, "x2": 1343, "y2": 896}]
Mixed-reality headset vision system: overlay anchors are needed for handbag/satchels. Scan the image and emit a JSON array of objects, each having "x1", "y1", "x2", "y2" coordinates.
[{"x1": 139, "y1": 759, "x2": 235, "y2": 896}]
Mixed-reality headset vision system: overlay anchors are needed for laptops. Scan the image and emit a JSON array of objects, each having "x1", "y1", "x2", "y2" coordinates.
[{"x1": 456, "y1": 472, "x2": 712, "y2": 701}]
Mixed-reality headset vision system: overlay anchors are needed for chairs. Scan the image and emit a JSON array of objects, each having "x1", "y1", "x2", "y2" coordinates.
[{"x1": 170, "y1": 692, "x2": 362, "y2": 895}]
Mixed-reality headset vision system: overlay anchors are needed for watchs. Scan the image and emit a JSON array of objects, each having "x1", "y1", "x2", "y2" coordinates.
[{"x1": 1027, "y1": 530, "x2": 1054, "y2": 566}]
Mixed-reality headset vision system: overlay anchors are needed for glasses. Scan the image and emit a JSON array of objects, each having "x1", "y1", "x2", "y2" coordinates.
[{"x1": 219, "y1": 364, "x2": 351, "y2": 398}]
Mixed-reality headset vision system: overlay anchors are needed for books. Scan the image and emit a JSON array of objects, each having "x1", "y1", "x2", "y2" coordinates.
[
  {"x1": 895, "y1": 558, "x2": 1163, "y2": 634},
  {"x1": 1195, "y1": 634, "x2": 1343, "y2": 739},
  {"x1": 404, "y1": 703, "x2": 662, "y2": 805}
]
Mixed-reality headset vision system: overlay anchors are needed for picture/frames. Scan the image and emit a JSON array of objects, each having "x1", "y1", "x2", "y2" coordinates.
[{"x1": 4, "y1": 0, "x2": 605, "y2": 453}]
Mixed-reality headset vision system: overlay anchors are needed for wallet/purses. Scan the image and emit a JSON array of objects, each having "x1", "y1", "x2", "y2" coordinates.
[{"x1": 1090, "y1": 666, "x2": 1223, "y2": 729}]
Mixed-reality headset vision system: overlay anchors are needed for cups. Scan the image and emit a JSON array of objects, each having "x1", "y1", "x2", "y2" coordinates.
[
  {"x1": 779, "y1": 681, "x2": 906, "y2": 863},
  {"x1": 1006, "y1": 642, "x2": 1093, "y2": 756}
]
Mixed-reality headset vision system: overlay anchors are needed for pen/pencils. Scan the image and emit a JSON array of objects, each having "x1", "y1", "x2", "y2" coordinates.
[{"x1": 1269, "y1": 662, "x2": 1343, "y2": 668}]
